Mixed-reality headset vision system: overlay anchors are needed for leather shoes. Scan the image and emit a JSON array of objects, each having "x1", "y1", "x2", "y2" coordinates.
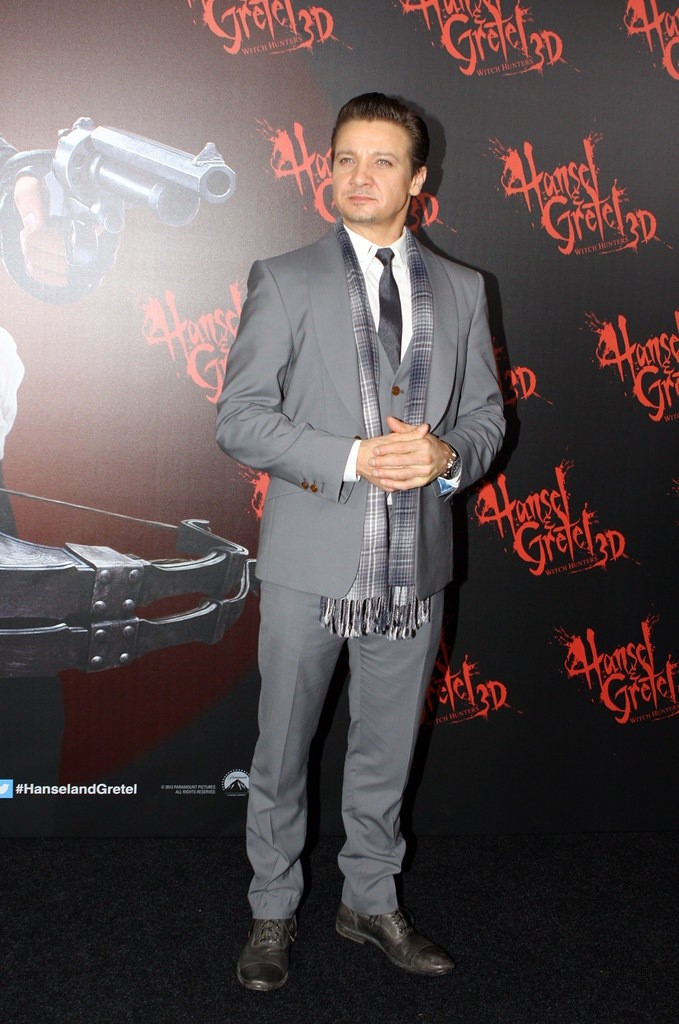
[
  {"x1": 236, "y1": 914, "x2": 297, "y2": 992},
  {"x1": 335, "y1": 902, "x2": 455, "y2": 976}
]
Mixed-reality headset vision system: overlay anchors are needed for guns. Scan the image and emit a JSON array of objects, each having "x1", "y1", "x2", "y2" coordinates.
[{"x1": 33, "y1": 115, "x2": 237, "y2": 272}]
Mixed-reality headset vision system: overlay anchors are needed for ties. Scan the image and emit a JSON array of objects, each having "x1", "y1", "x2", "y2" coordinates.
[{"x1": 377, "y1": 247, "x2": 402, "y2": 374}]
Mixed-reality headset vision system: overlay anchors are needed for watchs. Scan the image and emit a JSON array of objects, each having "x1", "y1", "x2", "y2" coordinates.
[{"x1": 442, "y1": 442, "x2": 462, "y2": 481}]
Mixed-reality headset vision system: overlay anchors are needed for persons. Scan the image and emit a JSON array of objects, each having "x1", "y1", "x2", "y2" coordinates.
[{"x1": 213, "y1": 88, "x2": 514, "y2": 993}]
